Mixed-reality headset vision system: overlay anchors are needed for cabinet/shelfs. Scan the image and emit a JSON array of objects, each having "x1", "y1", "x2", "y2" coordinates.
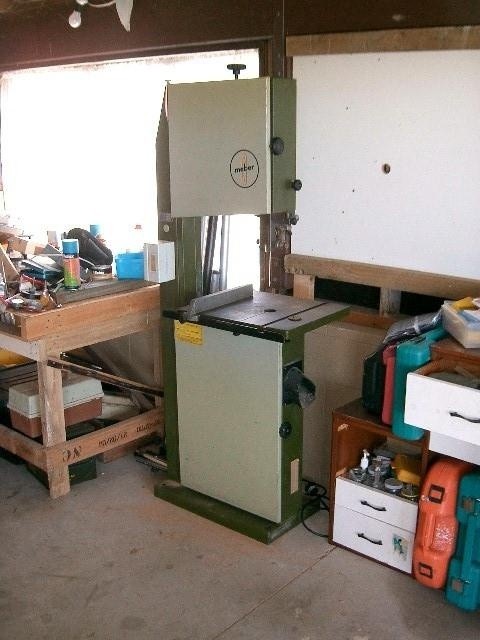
[
  {"x1": 404, "y1": 334, "x2": 480, "y2": 612},
  {"x1": 329, "y1": 393, "x2": 432, "y2": 570}
]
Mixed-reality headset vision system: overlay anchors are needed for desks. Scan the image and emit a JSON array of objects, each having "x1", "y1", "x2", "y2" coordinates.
[{"x1": 0, "y1": 274, "x2": 167, "y2": 500}]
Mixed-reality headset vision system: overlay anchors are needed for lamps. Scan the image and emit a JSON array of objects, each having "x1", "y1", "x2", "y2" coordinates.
[{"x1": 66, "y1": 0, "x2": 90, "y2": 29}]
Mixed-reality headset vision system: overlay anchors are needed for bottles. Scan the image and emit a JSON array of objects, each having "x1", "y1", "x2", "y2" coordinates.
[
  {"x1": 40, "y1": 290, "x2": 50, "y2": 308},
  {"x1": 351, "y1": 463, "x2": 419, "y2": 502},
  {"x1": 62, "y1": 238, "x2": 81, "y2": 291}
]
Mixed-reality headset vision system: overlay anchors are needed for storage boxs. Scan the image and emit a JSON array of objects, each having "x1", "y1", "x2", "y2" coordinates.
[
  {"x1": 114, "y1": 252, "x2": 145, "y2": 278},
  {"x1": 442, "y1": 298, "x2": 480, "y2": 351},
  {"x1": 0, "y1": 360, "x2": 150, "y2": 490},
  {"x1": 143, "y1": 238, "x2": 177, "y2": 285}
]
